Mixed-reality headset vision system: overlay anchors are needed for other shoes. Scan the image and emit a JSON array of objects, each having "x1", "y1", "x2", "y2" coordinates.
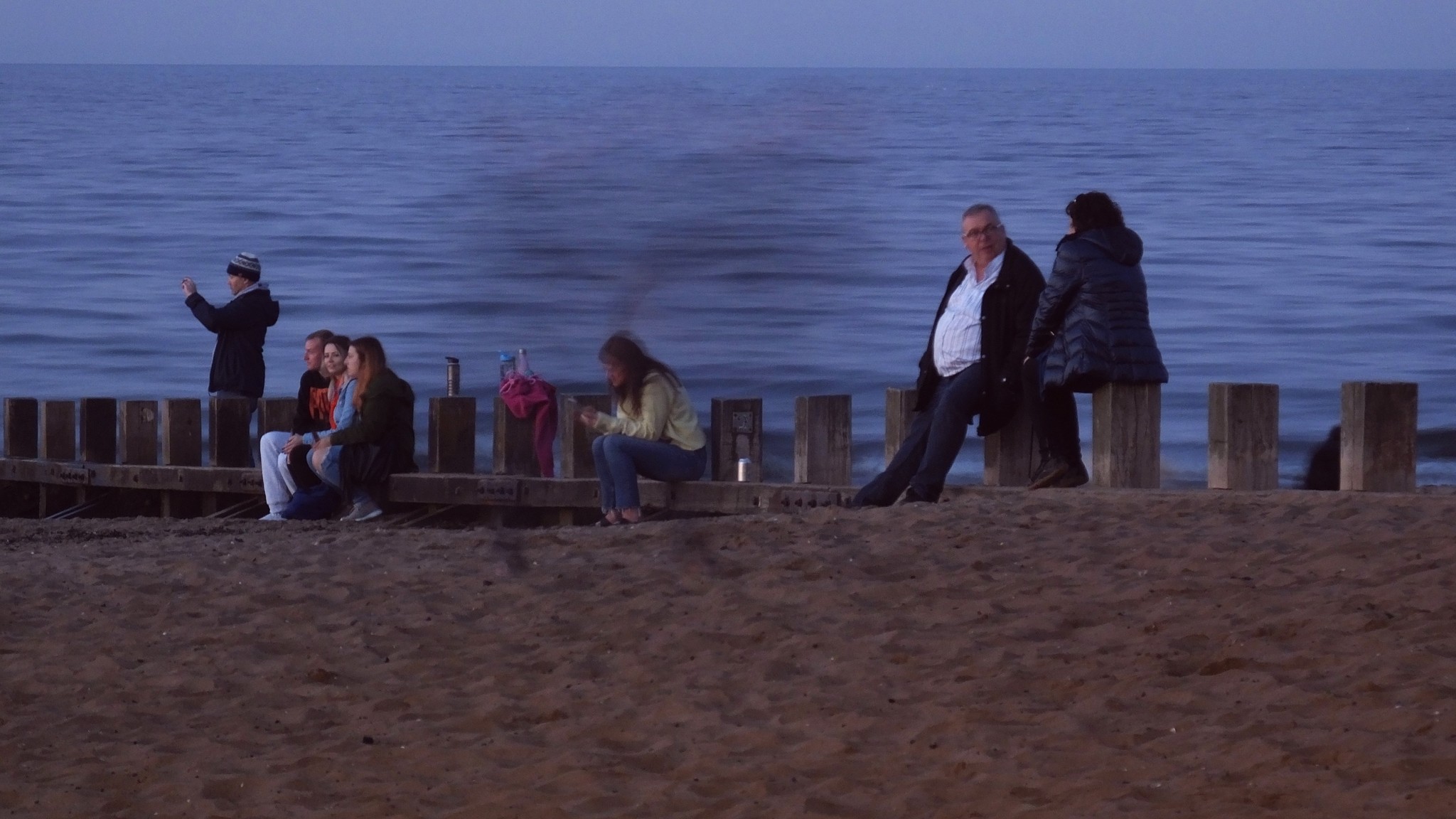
[
  {"x1": 1027, "y1": 454, "x2": 1089, "y2": 490},
  {"x1": 899, "y1": 486, "x2": 938, "y2": 505},
  {"x1": 258, "y1": 511, "x2": 288, "y2": 520}
]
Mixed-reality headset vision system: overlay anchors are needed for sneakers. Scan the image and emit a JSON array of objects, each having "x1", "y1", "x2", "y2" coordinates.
[{"x1": 340, "y1": 496, "x2": 383, "y2": 522}]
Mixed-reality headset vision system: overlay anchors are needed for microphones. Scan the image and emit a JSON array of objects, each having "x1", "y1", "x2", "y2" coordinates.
[{"x1": 568, "y1": 399, "x2": 589, "y2": 411}]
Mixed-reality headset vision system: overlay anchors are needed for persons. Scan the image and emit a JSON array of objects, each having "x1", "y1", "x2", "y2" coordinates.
[
  {"x1": 848, "y1": 202, "x2": 1047, "y2": 508},
  {"x1": 582, "y1": 333, "x2": 707, "y2": 527},
  {"x1": 183, "y1": 251, "x2": 280, "y2": 468},
  {"x1": 1024, "y1": 190, "x2": 1168, "y2": 490},
  {"x1": 260, "y1": 329, "x2": 416, "y2": 521}
]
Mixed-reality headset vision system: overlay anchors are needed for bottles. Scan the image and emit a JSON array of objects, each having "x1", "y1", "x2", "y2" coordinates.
[
  {"x1": 445, "y1": 356, "x2": 460, "y2": 397},
  {"x1": 517, "y1": 349, "x2": 529, "y2": 374},
  {"x1": 499, "y1": 354, "x2": 516, "y2": 382}
]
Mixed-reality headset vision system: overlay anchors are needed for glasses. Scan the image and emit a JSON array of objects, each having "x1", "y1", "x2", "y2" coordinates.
[
  {"x1": 1074, "y1": 194, "x2": 1086, "y2": 206},
  {"x1": 963, "y1": 222, "x2": 1002, "y2": 242}
]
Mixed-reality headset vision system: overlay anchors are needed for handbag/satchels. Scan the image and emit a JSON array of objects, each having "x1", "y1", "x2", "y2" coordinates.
[{"x1": 280, "y1": 480, "x2": 347, "y2": 521}]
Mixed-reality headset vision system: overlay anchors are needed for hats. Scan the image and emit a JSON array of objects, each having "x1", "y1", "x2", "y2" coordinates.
[{"x1": 227, "y1": 252, "x2": 261, "y2": 282}]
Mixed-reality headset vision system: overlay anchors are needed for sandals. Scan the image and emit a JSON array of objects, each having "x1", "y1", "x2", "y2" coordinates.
[{"x1": 594, "y1": 514, "x2": 647, "y2": 527}]
